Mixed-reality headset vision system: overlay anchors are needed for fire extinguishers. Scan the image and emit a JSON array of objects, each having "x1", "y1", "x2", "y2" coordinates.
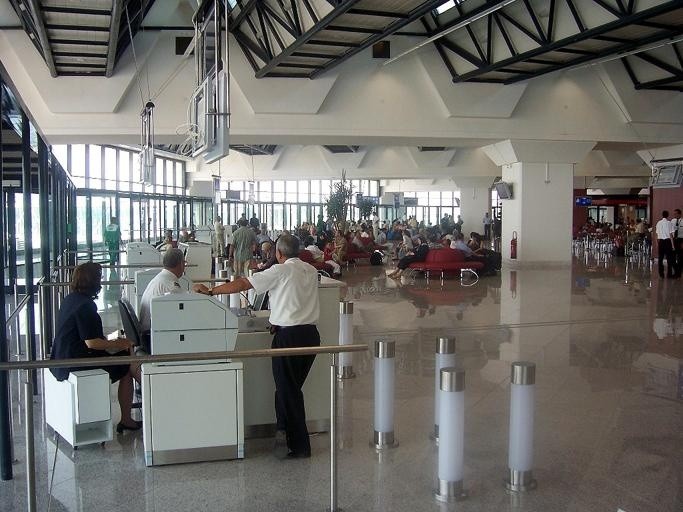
[
  {"x1": 511, "y1": 231, "x2": 517, "y2": 259},
  {"x1": 510, "y1": 271, "x2": 516, "y2": 299}
]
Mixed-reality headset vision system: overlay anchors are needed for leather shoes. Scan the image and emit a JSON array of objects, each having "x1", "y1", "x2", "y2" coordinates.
[
  {"x1": 286, "y1": 446, "x2": 311, "y2": 457},
  {"x1": 116, "y1": 418, "x2": 142, "y2": 434}
]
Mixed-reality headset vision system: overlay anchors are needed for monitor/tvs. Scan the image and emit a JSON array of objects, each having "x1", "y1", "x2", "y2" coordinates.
[
  {"x1": 494, "y1": 181, "x2": 511, "y2": 200},
  {"x1": 192, "y1": 69, "x2": 229, "y2": 164},
  {"x1": 653, "y1": 165, "x2": 683, "y2": 189},
  {"x1": 252, "y1": 290, "x2": 269, "y2": 311},
  {"x1": 138, "y1": 148, "x2": 154, "y2": 187},
  {"x1": 575, "y1": 196, "x2": 592, "y2": 207}
]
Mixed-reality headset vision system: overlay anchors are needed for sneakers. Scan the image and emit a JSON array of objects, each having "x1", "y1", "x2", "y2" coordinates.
[
  {"x1": 388, "y1": 273, "x2": 401, "y2": 279},
  {"x1": 659, "y1": 273, "x2": 683, "y2": 280}
]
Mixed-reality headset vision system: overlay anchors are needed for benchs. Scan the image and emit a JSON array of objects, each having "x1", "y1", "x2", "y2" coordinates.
[{"x1": 237, "y1": 220, "x2": 501, "y2": 289}]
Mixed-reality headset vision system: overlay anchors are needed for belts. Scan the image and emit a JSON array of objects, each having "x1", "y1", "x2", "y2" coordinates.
[{"x1": 277, "y1": 325, "x2": 290, "y2": 331}]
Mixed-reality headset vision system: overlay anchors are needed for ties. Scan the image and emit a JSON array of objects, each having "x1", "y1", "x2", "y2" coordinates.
[{"x1": 674, "y1": 218, "x2": 680, "y2": 239}]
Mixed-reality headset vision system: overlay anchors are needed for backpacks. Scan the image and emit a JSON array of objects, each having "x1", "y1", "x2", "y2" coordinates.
[{"x1": 370, "y1": 252, "x2": 383, "y2": 265}]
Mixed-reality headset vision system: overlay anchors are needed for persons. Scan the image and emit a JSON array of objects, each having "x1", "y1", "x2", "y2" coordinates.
[
  {"x1": 103, "y1": 216, "x2": 122, "y2": 266},
  {"x1": 139, "y1": 250, "x2": 198, "y2": 355},
  {"x1": 652, "y1": 276, "x2": 675, "y2": 343},
  {"x1": 573, "y1": 216, "x2": 652, "y2": 255},
  {"x1": 350, "y1": 278, "x2": 501, "y2": 322},
  {"x1": 670, "y1": 277, "x2": 683, "y2": 343},
  {"x1": 656, "y1": 210, "x2": 676, "y2": 278},
  {"x1": 47, "y1": 259, "x2": 140, "y2": 435},
  {"x1": 192, "y1": 235, "x2": 320, "y2": 459},
  {"x1": 670, "y1": 208, "x2": 683, "y2": 275},
  {"x1": 102, "y1": 265, "x2": 122, "y2": 309}
]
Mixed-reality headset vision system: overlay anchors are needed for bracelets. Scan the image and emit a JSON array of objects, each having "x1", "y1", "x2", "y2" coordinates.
[{"x1": 207, "y1": 287, "x2": 213, "y2": 297}]
